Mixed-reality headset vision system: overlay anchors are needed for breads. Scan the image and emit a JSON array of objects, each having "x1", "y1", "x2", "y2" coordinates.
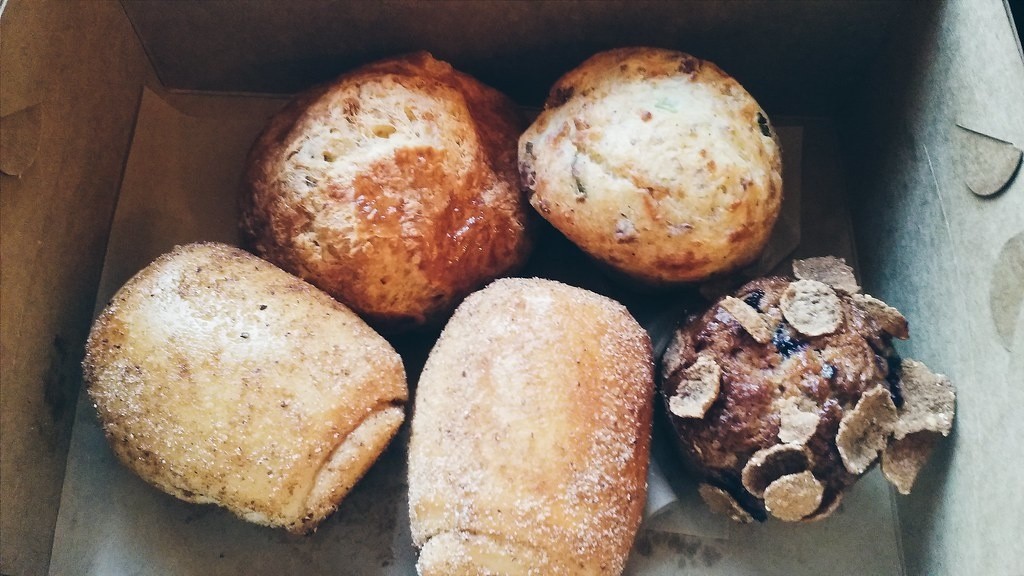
[
  {"x1": 237, "y1": 45, "x2": 543, "y2": 325},
  {"x1": 81, "y1": 240, "x2": 410, "y2": 535},
  {"x1": 404, "y1": 277, "x2": 656, "y2": 576},
  {"x1": 519, "y1": 44, "x2": 783, "y2": 285}
]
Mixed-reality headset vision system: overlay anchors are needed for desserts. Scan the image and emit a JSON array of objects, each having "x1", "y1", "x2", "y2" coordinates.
[{"x1": 649, "y1": 253, "x2": 958, "y2": 524}]
